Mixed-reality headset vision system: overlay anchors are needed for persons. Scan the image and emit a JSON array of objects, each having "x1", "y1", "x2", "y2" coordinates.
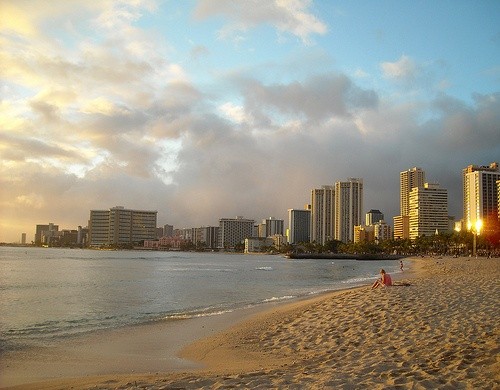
[
  {"x1": 399, "y1": 260, "x2": 404, "y2": 271},
  {"x1": 371, "y1": 269, "x2": 392, "y2": 289},
  {"x1": 417, "y1": 247, "x2": 500, "y2": 262}
]
